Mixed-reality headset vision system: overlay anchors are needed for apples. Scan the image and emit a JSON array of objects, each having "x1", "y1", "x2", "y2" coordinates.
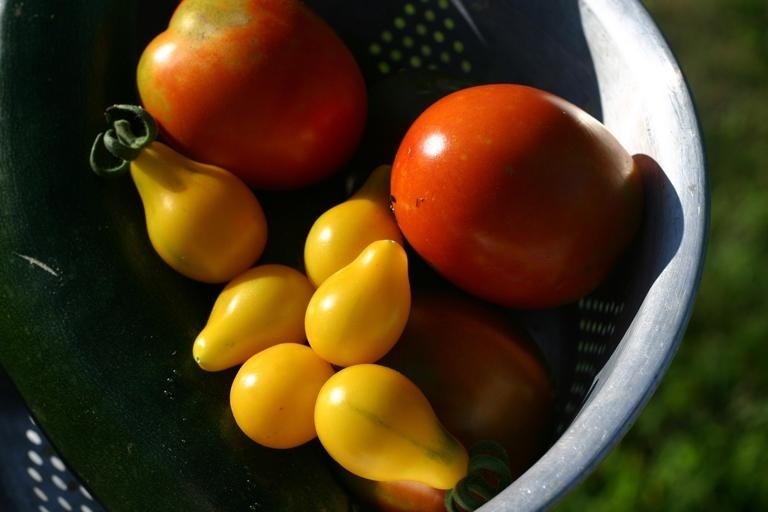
[
  {"x1": 136, "y1": 0, "x2": 370, "y2": 189},
  {"x1": 331, "y1": 298, "x2": 551, "y2": 508},
  {"x1": 389, "y1": 83, "x2": 647, "y2": 313}
]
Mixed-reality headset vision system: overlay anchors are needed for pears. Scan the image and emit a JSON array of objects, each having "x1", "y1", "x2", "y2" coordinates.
[
  {"x1": 192, "y1": 267, "x2": 314, "y2": 367},
  {"x1": 302, "y1": 241, "x2": 409, "y2": 367},
  {"x1": 124, "y1": 138, "x2": 263, "y2": 280},
  {"x1": 298, "y1": 162, "x2": 392, "y2": 289},
  {"x1": 315, "y1": 367, "x2": 515, "y2": 512},
  {"x1": 226, "y1": 341, "x2": 334, "y2": 450}
]
